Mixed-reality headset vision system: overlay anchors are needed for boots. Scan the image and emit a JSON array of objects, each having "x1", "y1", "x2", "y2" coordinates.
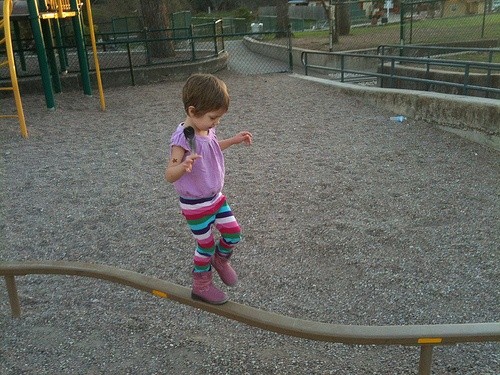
[
  {"x1": 211, "y1": 245, "x2": 237, "y2": 286},
  {"x1": 191, "y1": 268, "x2": 229, "y2": 304}
]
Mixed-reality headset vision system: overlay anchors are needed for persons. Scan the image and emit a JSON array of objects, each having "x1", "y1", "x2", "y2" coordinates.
[{"x1": 165, "y1": 73, "x2": 252, "y2": 305}]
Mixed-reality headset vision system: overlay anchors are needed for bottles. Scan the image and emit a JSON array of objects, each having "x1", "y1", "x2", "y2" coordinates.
[{"x1": 390, "y1": 116, "x2": 407, "y2": 122}]
